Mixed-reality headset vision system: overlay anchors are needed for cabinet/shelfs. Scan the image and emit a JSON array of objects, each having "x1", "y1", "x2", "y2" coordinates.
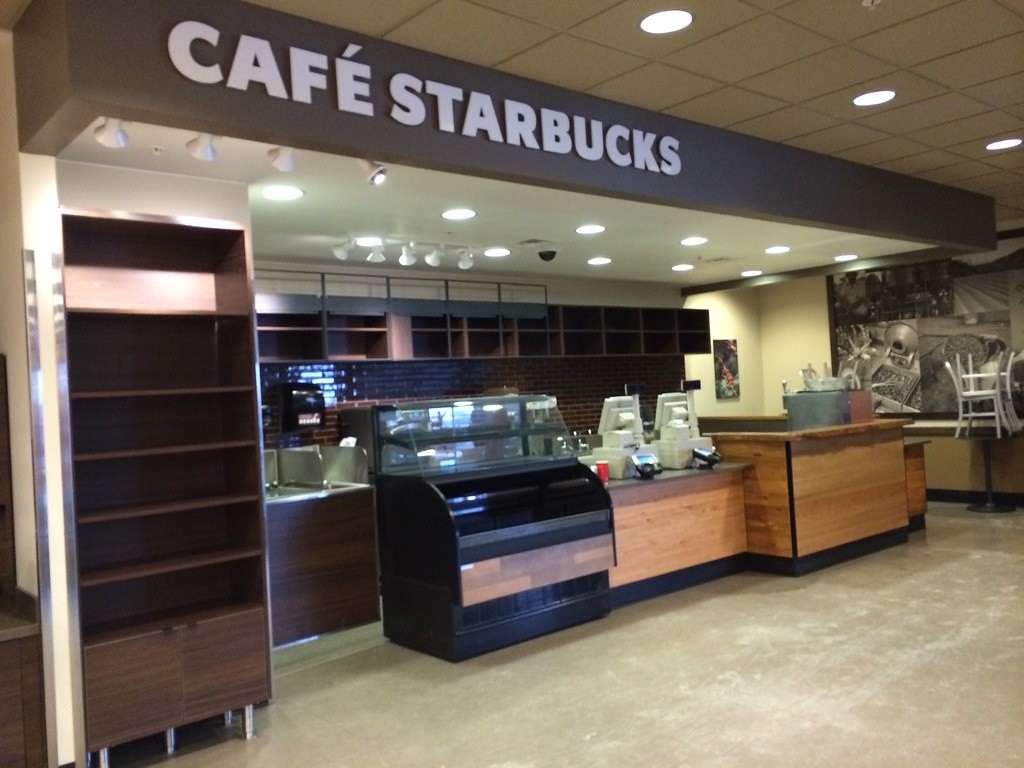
[
  {"x1": 370, "y1": 395, "x2": 618, "y2": 663},
  {"x1": 256, "y1": 293, "x2": 712, "y2": 364},
  {"x1": 39, "y1": 207, "x2": 273, "y2": 768}
]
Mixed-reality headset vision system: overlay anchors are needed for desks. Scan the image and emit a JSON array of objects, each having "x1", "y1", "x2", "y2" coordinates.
[{"x1": 596, "y1": 415, "x2": 934, "y2": 607}]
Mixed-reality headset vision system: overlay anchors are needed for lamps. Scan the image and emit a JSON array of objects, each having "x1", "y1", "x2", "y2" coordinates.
[{"x1": 93, "y1": 116, "x2": 473, "y2": 273}]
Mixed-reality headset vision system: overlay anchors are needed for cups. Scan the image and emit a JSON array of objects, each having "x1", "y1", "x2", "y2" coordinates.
[{"x1": 596, "y1": 460, "x2": 609, "y2": 486}]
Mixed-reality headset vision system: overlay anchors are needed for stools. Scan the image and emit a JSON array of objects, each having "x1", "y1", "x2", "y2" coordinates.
[
  {"x1": 945, "y1": 435, "x2": 1022, "y2": 514},
  {"x1": 945, "y1": 352, "x2": 1022, "y2": 440}
]
